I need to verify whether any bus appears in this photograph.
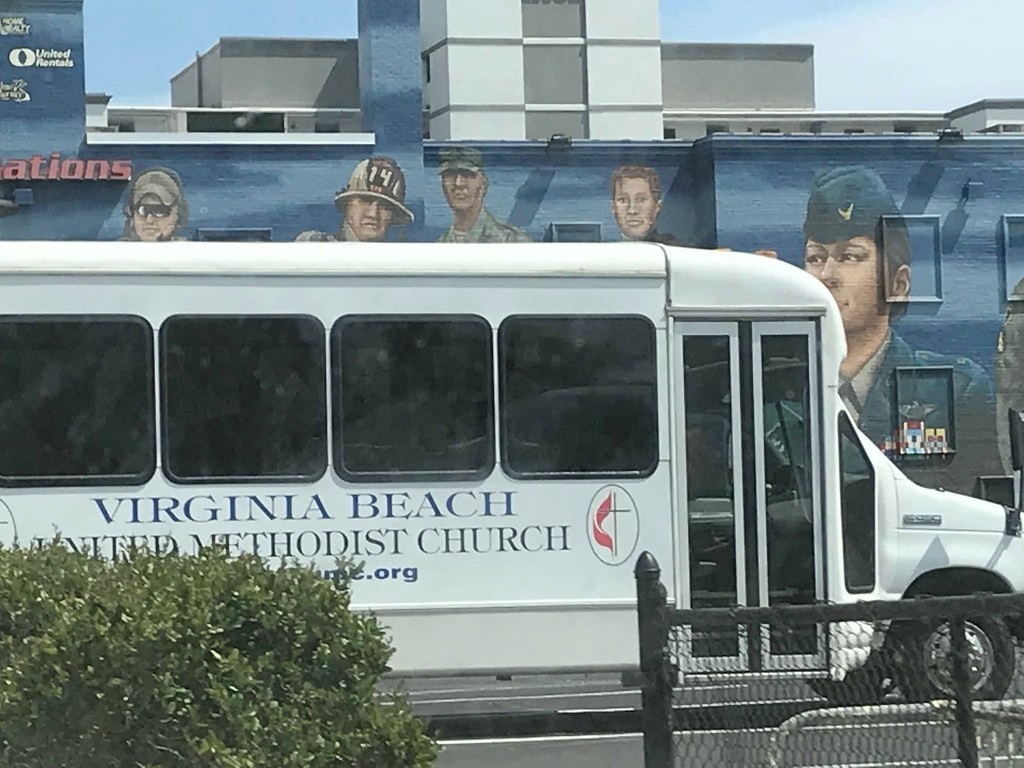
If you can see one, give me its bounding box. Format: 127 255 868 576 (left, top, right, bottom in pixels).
0 237 1024 724
